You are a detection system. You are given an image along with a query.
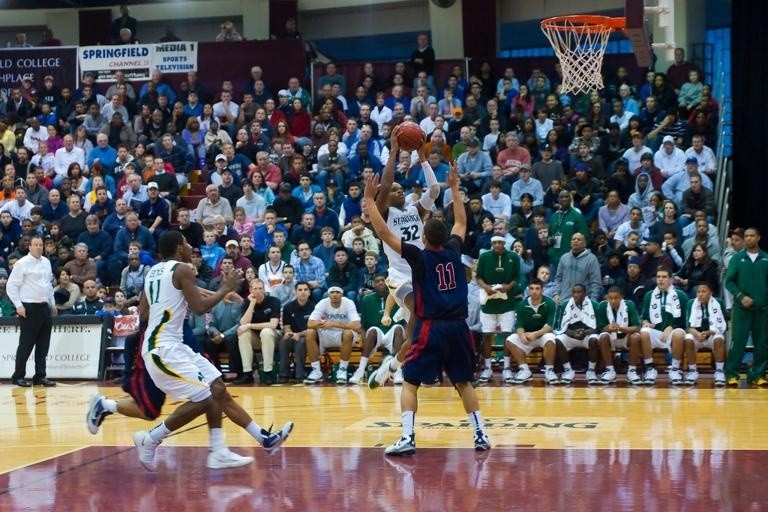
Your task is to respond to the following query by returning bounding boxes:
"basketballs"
[397,122,424,151]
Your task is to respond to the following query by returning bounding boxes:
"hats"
[729,228,745,239]
[643,235,662,244]
[686,156,697,164]
[148,182,158,189]
[412,179,422,186]
[520,164,531,172]
[226,240,239,248]
[663,135,674,145]
[465,140,479,146]
[215,154,226,162]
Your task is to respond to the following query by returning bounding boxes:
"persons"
[86,262,296,457]
[132,231,256,470]
[361,159,492,456]
[0,5,768,388]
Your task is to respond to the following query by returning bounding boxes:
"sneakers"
[233,375,254,385]
[475,431,490,451]
[261,422,295,457]
[383,433,415,455]
[368,354,404,389]
[133,430,157,472]
[86,393,112,434]
[752,378,768,385]
[350,371,366,382]
[263,370,347,384]
[479,366,739,386]
[206,449,254,469]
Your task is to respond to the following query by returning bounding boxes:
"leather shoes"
[33,378,55,386]
[12,375,30,387]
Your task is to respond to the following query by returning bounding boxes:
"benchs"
[85,342,728,385]
